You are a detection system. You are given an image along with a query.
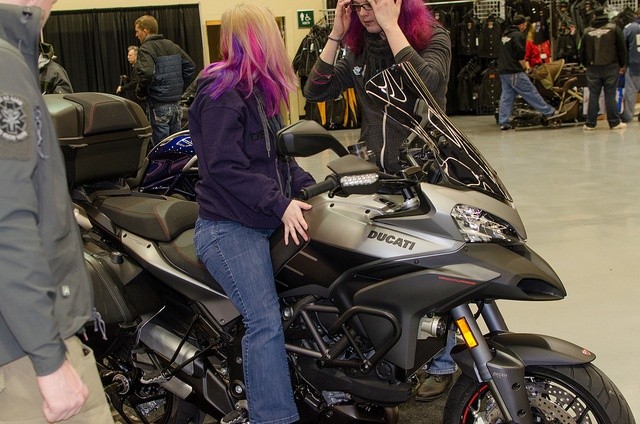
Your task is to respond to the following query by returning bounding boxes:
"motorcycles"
[36,88,639,421]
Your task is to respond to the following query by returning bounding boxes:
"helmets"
[138,135,195,193]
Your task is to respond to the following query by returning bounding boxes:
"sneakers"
[619,112,628,123]
[612,122,626,129]
[501,126,515,130]
[582,124,595,130]
[546,109,567,120]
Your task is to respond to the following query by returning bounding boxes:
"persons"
[133,14,196,144]
[302,0,452,176]
[495,15,567,130]
[621,8,640,123]
[579,6,628,129]
[525,16,551,72]
[116,45,147,114]
[186,3,312,424]
[1,0,117,423]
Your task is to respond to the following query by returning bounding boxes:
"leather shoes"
[415,374,453,402]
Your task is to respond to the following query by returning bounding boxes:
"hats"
[595,7,606,15]
[513,14,530,25]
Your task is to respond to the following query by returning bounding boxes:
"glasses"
[350,4,373,12]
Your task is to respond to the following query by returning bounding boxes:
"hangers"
[462,9,475,23]
[481,60,498,75]
[305,21,327,40]
[456,58,478,79]
